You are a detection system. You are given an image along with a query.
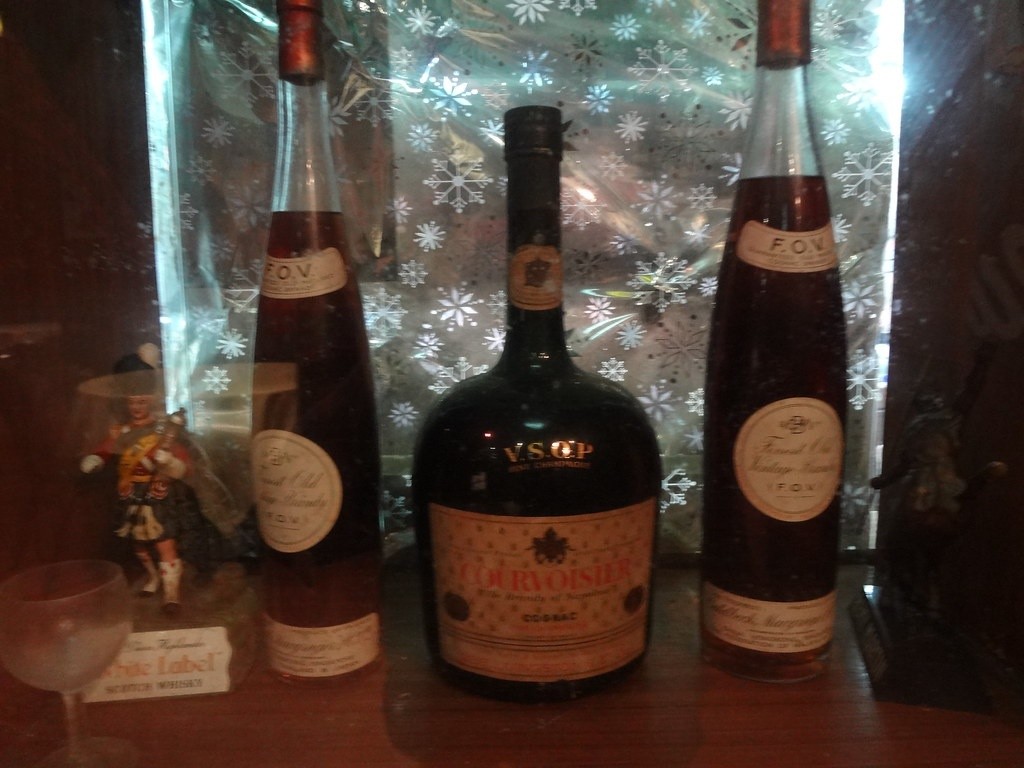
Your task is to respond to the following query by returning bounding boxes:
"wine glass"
[0,562,140,768]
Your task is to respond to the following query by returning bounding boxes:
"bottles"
[412,108,662,699]
[702,0,849,681]
[251,0,390,685]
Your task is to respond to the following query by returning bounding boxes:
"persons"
[79,351,191,614]
